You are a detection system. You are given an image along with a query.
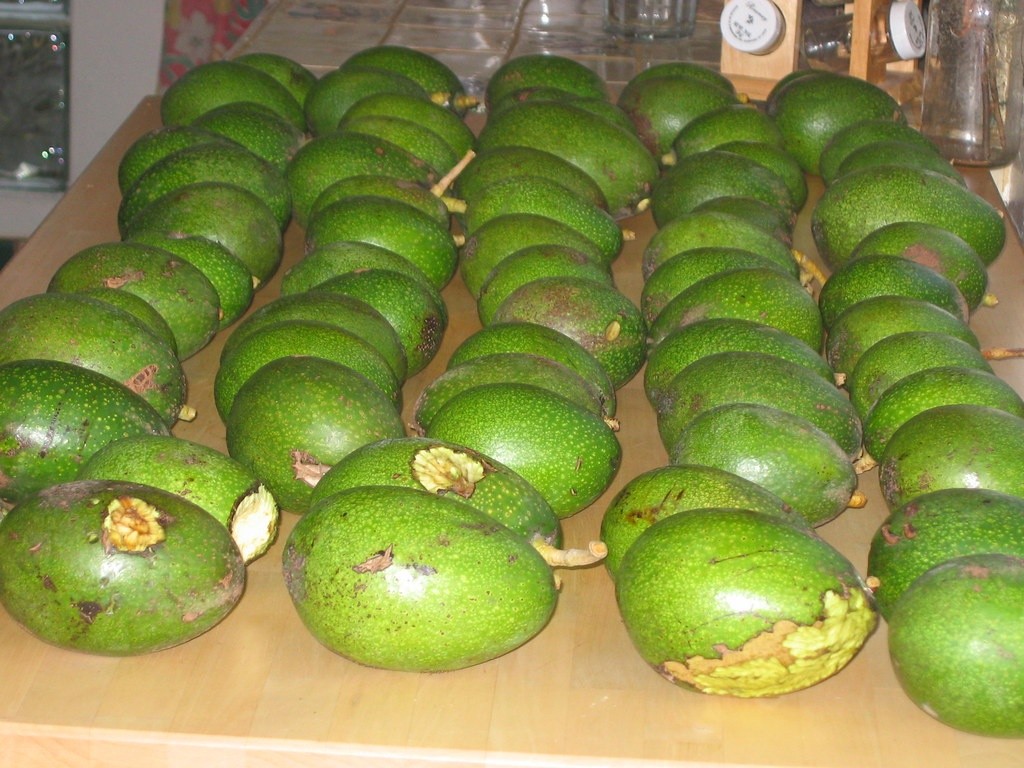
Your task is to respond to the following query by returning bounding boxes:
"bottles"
[795,0,925,75]
[919,0,1024,169]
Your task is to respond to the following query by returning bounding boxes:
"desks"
[0,86,1024,768]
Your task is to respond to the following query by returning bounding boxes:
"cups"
[608,0,698,43]
